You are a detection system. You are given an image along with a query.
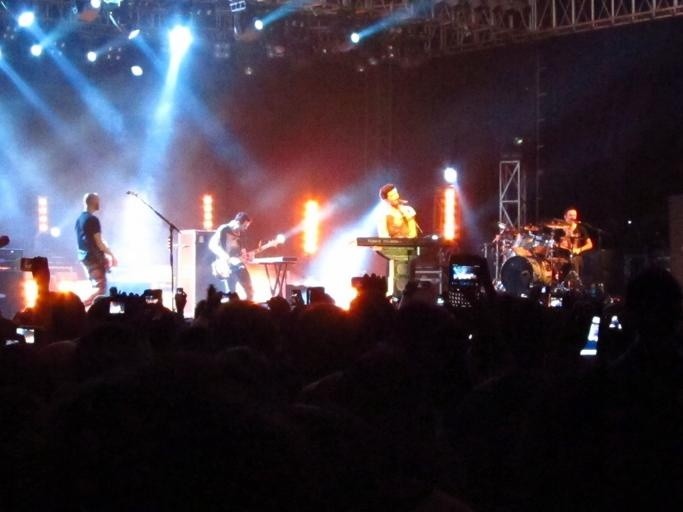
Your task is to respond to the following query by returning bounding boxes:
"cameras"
[218,294,232,304]
[392,296,401,303]
[435,296,446,304]
[550,295,564,308]
[144,294,160,305]
[606,313,624,331]
[14,326,36,346]
[107,298,127,316]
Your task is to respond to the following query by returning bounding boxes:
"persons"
[207,212,268,303]
[558,207,596,289]
[74,192,118,307]
[378,183,420,304]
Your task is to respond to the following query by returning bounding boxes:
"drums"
[511,234,541,257]
[529,235,555,256]
[500,256,559,297]
[546,246,571,264]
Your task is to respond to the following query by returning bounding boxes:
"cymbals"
[524,226,539,231]
[546,224,571,229]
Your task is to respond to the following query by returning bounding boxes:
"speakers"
[178,229,226,321]
[286,285,324,310]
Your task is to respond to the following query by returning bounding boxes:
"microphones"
[399,199,408,204]
[571,218,581,223]
[126,190,138,197]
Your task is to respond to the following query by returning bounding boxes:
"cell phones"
[578,314,603,358]
[352,277,371,287]
[21,258,34,271]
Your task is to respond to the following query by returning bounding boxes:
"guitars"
[211,234,286,280]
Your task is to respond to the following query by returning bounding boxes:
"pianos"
[356,236,460,247]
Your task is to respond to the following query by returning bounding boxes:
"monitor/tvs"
[449,260,485,287]
